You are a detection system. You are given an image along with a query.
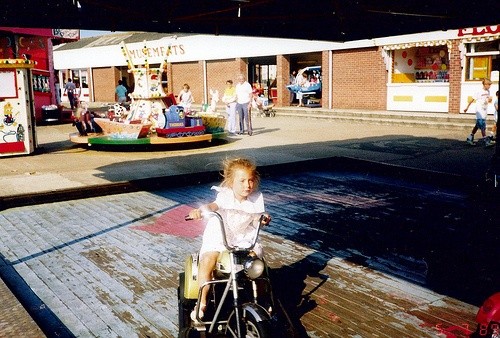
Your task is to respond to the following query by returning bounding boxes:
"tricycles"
[177,211,273,338]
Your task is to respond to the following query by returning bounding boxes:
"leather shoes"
[190,302,207,321]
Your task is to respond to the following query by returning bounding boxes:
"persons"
[222,79,238,135]
[177,83,195,111]
[463,77,496,147]
[115,80,128,103]
[64,78,78,109]
[233,73,253,133]
[491,89,500,140]
[188,156,273,323]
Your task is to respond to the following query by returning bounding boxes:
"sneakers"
[482,138,496,147]
[466,138,476,145]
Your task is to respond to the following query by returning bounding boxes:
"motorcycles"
[252,98,275,118]
